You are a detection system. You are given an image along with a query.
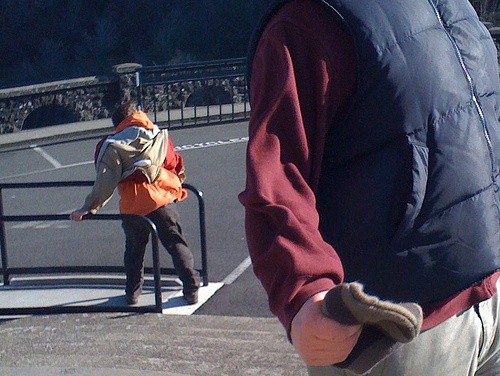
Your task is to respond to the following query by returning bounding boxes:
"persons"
[67,101,203,305]
[235,0,500,374]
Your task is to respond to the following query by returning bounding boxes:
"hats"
[323,281,425,345]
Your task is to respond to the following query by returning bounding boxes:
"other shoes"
[182,287,199,305]
[124,293,139,305]
